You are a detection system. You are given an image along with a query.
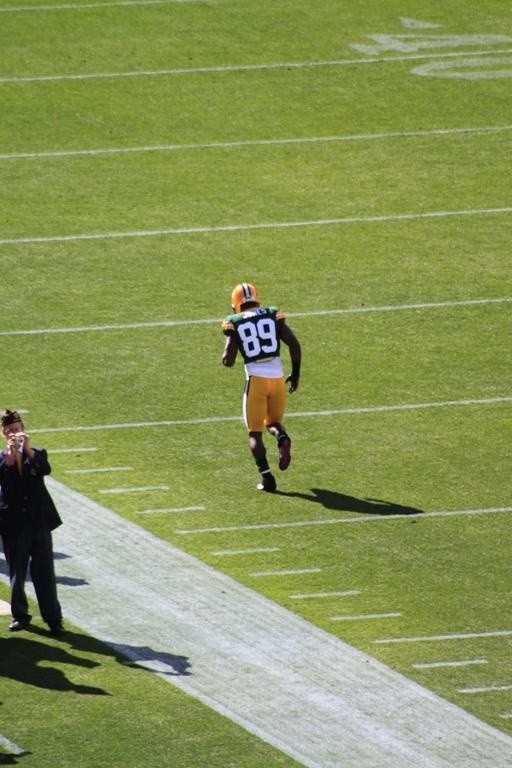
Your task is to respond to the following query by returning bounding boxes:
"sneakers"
[257,477,275,492]
[278,435,291,470]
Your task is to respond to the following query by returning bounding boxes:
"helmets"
[232,283,259,312]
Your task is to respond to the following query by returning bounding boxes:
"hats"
[1,410,20,426]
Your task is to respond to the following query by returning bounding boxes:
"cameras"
[14,432,25,446]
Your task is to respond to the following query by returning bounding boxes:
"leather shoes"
[8,615,32,630]
[48,618,63,635]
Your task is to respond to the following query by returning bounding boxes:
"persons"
[0,409,72,642]
[222,282,303,493]
